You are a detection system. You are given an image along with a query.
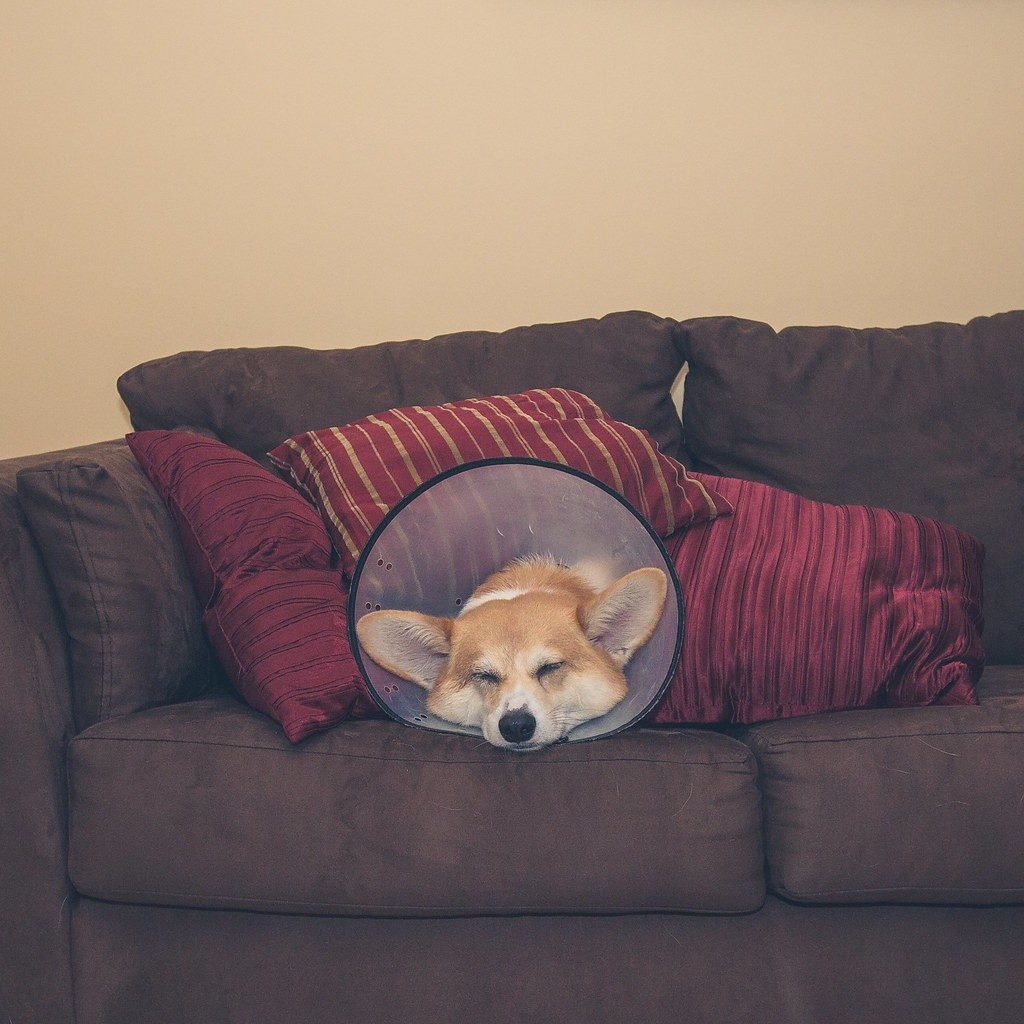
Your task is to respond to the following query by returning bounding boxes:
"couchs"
[0,301,1023,1024]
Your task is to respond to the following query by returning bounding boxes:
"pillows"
[262,385,738,602]
[630,465,981,727]
[123,426,387,746]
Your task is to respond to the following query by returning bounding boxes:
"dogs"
[354,551,668,752]
